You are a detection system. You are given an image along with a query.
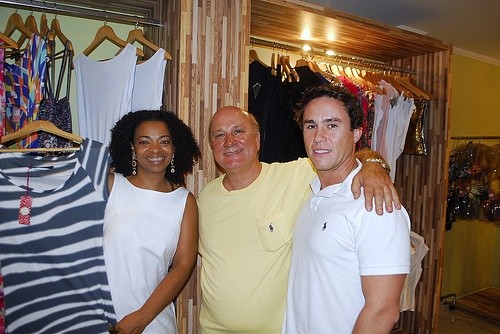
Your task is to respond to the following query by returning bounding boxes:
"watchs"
[363,157,391,173]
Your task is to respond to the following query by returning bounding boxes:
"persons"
[107,109,199,334]
[193,106,402,334]
[282,86,411,334]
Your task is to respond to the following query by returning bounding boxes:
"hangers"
[0,0,494,157]
